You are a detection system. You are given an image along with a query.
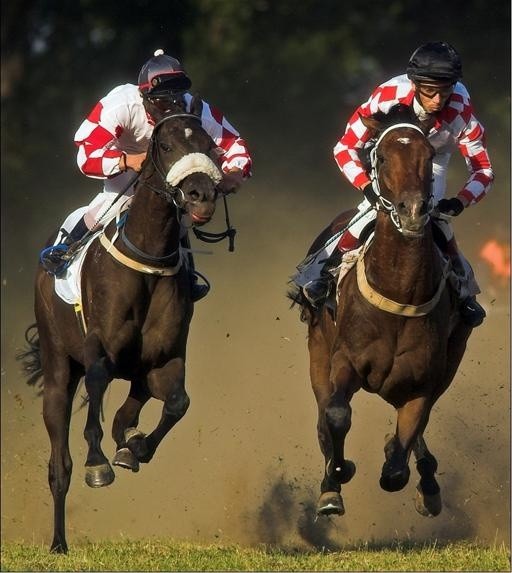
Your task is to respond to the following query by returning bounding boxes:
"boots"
[42,213,95,269]
[445,255,486,326]
[180,230,209,302]
[305,246,345,306]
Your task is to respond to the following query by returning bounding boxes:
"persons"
[300,42,499,330]
[38,49,252,302]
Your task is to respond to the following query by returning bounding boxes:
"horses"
[288,105,475,518]
[15,94,225,554]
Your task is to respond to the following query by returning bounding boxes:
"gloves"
[437,197,464,216]
[363,182,380,205]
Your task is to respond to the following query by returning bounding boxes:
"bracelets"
[123,153,129,172]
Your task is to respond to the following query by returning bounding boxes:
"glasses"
[414,81,457,98]
[147,97,185,110]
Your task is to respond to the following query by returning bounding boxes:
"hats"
[138,48,191,94]
[408,40,463,81]
